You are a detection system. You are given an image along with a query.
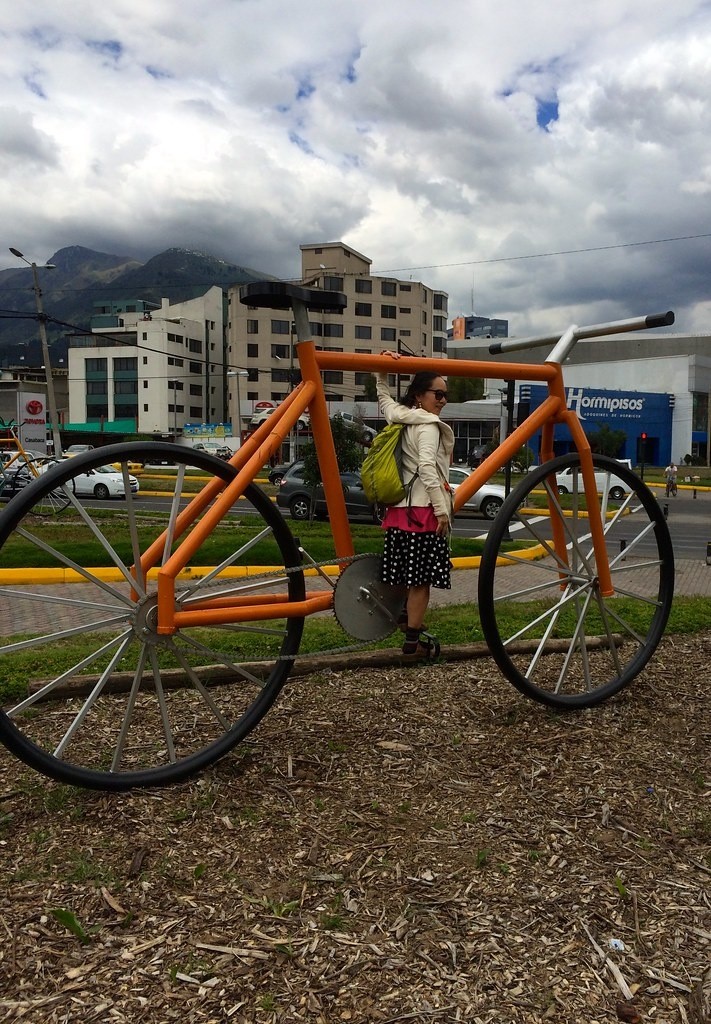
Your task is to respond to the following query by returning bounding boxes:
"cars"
[62,444,95,455]
[250,406,310,431]
[191,443,227,458]
[268,464,289,486]
[449,465,526,521]
[107,460,144,476]
[27,458,140,500]
[0,448,75,483]
[333,411,379,442]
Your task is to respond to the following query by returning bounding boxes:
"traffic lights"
[640,433,647,446]
[501,379,515,410]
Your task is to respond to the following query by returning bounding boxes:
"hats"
[669,462,674,466]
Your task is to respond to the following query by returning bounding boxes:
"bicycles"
[664,474,677,498]
[0,418,75,517]
[1,279,681,792]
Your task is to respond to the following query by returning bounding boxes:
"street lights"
[172,378,178,443]
[7,246,63,460]
[226,370,250,447]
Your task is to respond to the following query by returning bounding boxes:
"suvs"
[276,460,384,525]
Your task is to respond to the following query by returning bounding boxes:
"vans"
[556,458,633,500]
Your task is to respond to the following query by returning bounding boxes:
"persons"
[374,349,455,654]
[662,462,678,494]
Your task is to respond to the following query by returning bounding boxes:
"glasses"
[425,389,449,401]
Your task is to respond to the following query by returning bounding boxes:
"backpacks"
[359,423,423,529]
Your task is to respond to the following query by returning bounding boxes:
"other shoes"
[664,491,667,497]
[402,640,435,657]
